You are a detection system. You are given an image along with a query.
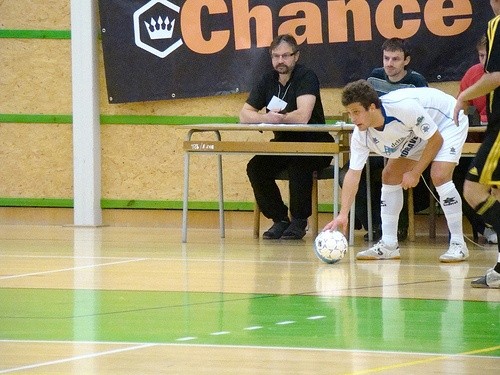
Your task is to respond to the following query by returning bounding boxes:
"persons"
[338,36,431,242]
[452,0,500,289]
[322,80,470,262]
[239,34,335,241]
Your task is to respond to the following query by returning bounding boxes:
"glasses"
[271,50,299,60]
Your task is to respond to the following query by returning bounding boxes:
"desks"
[175,124,342,244]
[342,123,488,246]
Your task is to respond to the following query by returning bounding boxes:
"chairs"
[253,113,479,241]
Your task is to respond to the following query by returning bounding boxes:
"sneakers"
[356,240,401,259]
[439,241,470,262]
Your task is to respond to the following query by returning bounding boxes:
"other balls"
[313,230,348,264]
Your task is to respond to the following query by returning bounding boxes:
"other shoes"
[364,225,408,243]
[484,227,498,244]
[263,218,290,239]
[281,222,308,239]
[471,265,500,289]
